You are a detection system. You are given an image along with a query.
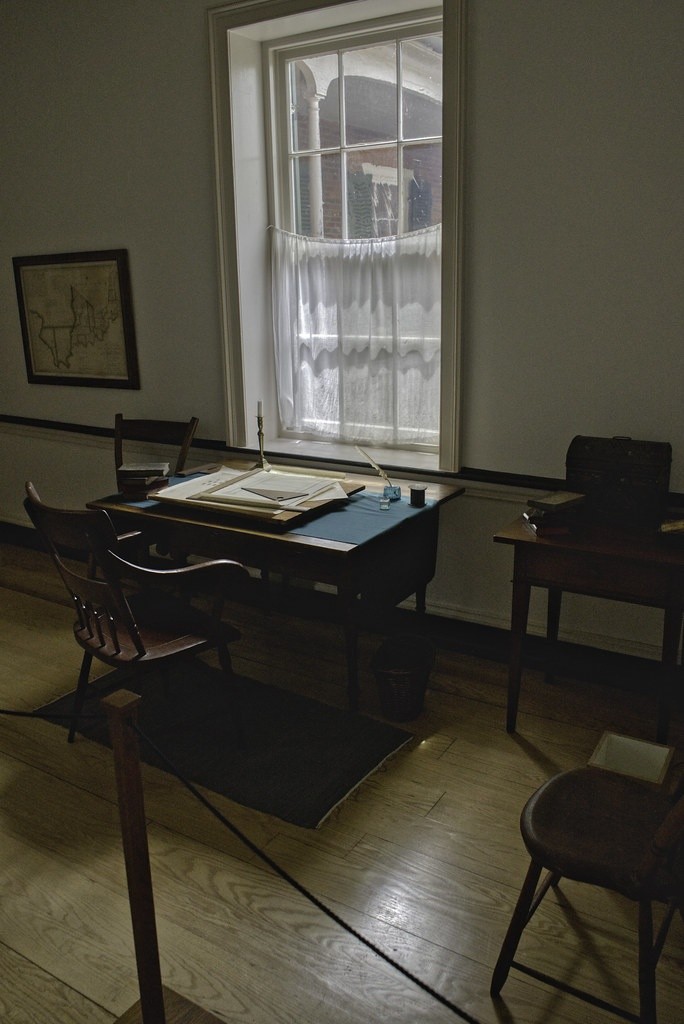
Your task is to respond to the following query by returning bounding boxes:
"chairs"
[115,413,200,486]
[487,768,684,1024]
[23,482,253,747]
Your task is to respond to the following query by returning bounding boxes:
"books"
[523,491,586,536]
[117,463,170,499]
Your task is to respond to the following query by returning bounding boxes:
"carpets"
[36,659,413,831]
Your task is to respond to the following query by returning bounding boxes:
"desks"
[88,457,466,746]
[492,505,684,734]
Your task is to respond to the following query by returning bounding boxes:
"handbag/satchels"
[564,435,673,507]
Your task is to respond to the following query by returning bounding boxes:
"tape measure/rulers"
[241,487,308,502]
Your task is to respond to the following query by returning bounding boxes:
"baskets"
[368,634,436,722]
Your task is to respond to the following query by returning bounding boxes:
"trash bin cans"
[370,633,437,721]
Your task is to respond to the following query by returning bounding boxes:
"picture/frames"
[11,249,141,391]
